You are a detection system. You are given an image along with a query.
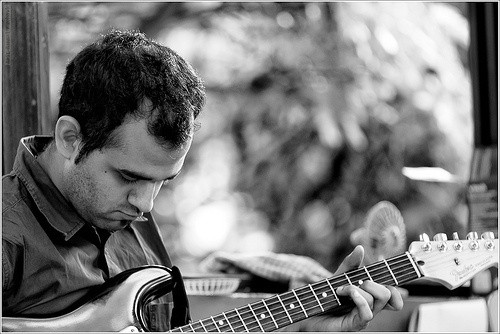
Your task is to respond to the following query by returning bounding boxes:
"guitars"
[19,232,499,333]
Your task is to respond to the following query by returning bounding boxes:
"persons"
[2,28,408,332]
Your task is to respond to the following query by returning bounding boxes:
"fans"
[348,199,410,266]
